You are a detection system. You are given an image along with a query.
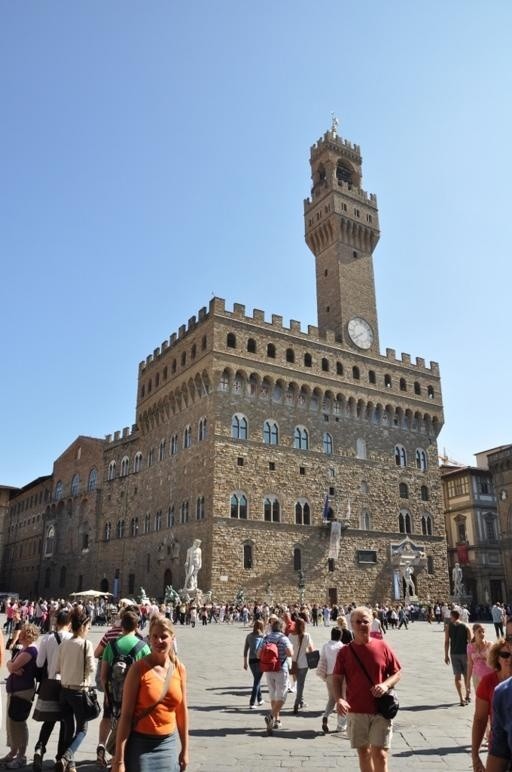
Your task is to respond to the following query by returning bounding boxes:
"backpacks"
[258,642,281,673]
[107,638,147,706]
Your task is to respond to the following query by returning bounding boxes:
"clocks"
[348,315,375,351]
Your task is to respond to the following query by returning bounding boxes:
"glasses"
[497,650,512,659]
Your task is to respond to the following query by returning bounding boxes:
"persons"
[402,561,415,596]
[453,562,462,595]
[184,539,202,588]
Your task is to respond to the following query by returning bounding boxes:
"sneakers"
[264,714,284,735]
[321,716,331,735]
[258,699,265,705]
[54,757,77,772]
[459,697,471,707]
[248,704,258,709]
[0,755,27,769]
[292,702,309,714]
[33,748,42,772]
[94,743,108,771]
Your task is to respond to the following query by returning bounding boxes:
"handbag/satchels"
[8,695,32,722]
[305,650,320,670]
[374,688,400,720]
[317,656,329,681]
[32,677,63,723]
[289,660,299,675]
[79,688,101,722]
